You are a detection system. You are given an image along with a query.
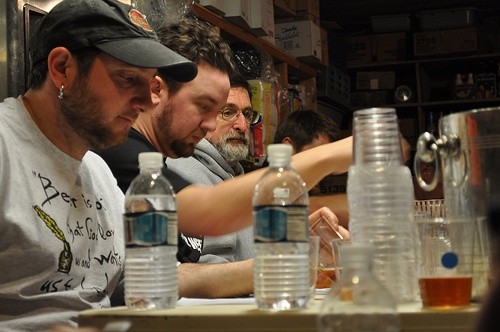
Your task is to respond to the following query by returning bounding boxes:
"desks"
[76,300,484,332]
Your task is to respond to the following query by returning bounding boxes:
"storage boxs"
[195,0,499,168]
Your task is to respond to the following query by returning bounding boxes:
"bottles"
[251,143,312,312]
[123,151,178,311]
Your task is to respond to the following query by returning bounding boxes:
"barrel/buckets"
[415,107,500,301]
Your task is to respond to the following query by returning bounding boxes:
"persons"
[93,18,411,261]
[0,0,312,332]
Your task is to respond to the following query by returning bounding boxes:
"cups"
[308,236,319,298]
[414,211,476,311]
[330,238,372,302]
[345,108,416,305]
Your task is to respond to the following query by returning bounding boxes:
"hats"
[28,0,197,83]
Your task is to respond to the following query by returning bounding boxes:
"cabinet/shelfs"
[344,6,500,151]
[191,4,320,174]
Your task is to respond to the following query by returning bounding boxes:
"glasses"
[219,108,262,125]
